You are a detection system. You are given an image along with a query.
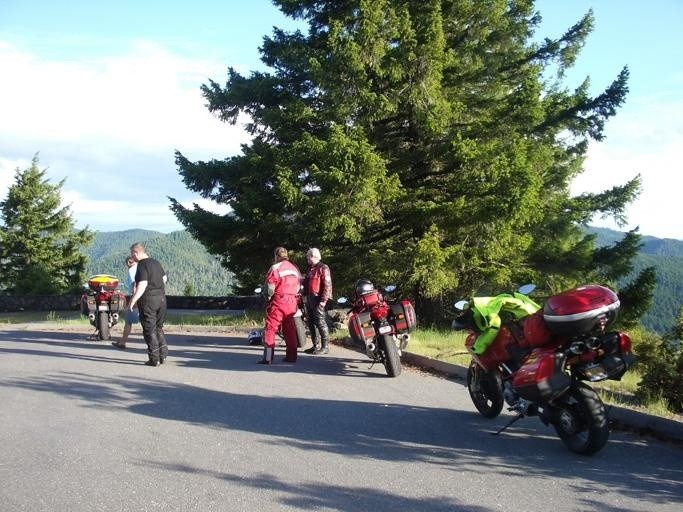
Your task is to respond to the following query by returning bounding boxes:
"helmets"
[248,330,262,345]
[356,279,374,297]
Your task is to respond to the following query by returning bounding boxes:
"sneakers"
[313,346,329,354]
[305,347,319,353]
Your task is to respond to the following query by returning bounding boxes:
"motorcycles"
[454,283,637,456]
[255,285,306,347]
[337,279,416,376]
[81,274,126,341]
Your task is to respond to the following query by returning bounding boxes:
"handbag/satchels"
[524,308,554,347]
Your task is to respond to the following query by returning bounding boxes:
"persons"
[302,248,333,354]
[112,256,138,349]
[129,243,168,367]
[257,247,302,364]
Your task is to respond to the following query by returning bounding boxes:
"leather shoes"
[282,357,296,363]
[111,341,125,349]
[145,358,166,366]
[256,359,271,364]
